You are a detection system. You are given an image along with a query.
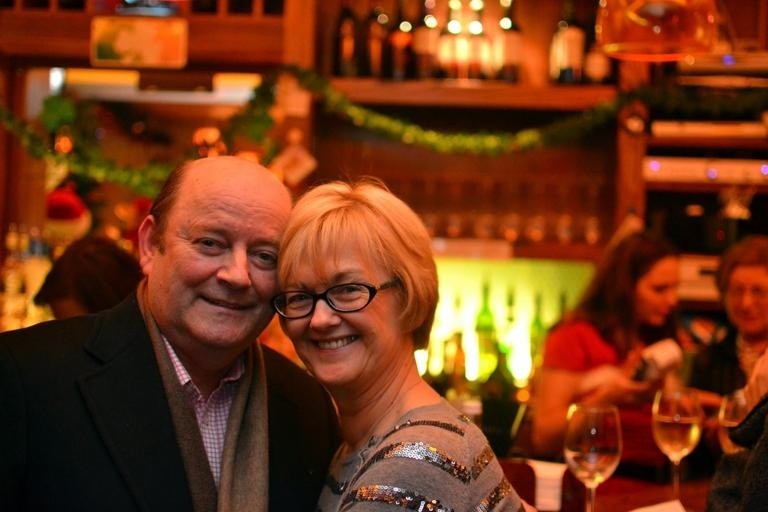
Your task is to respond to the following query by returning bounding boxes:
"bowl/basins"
[674,76,764,120]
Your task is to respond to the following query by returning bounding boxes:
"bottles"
[439,285,570,382]
[333,0,611,84]
[0,226,73,325]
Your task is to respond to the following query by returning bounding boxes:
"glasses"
[272,278,401,318]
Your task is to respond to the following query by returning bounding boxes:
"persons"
[680,236,768,512]
[0,156,342,512]
[530,234,732,456]
[277,180,525,512]
[34,235,140,320]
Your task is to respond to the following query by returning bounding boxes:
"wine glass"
[562,387,705,512]
